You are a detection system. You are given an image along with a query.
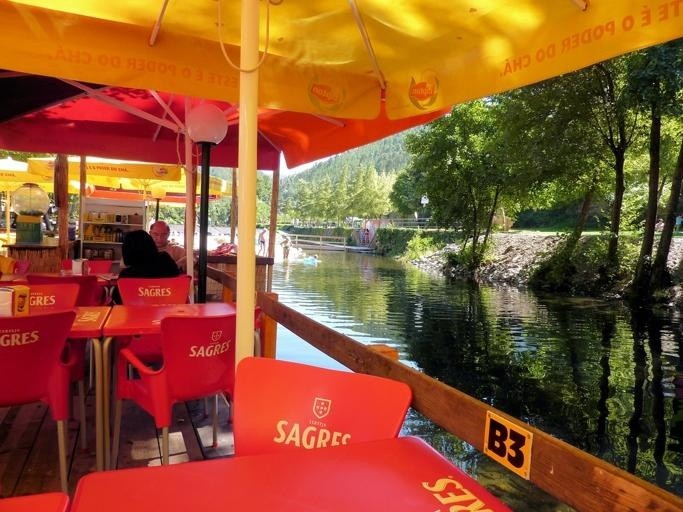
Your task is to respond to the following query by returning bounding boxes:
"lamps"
[185,103,228,304]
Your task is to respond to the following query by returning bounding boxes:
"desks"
[31,305,112,474]
[102,303,237,470]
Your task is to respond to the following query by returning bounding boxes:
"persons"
[279,235,292,260]
[358,227,364,245]
[148,221,197,272]
[303,254,318,261]
[256,228,267,257]
[363,228,370,245]
[110,230,181,304]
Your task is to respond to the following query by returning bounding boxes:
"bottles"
[115,210,121,224]
[68,216,76,240]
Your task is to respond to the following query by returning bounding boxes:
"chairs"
[231,355,414,455]
[110,305,263,470]
[1,260,193,308]
[2,310,86,494]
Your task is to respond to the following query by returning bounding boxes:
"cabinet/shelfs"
[80,196,146,264]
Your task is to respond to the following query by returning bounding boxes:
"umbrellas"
[0,0,683,369]
[28,156,232,199]
[1,70,453,305]
[0,156,94,243]
[86,185,222,224]
